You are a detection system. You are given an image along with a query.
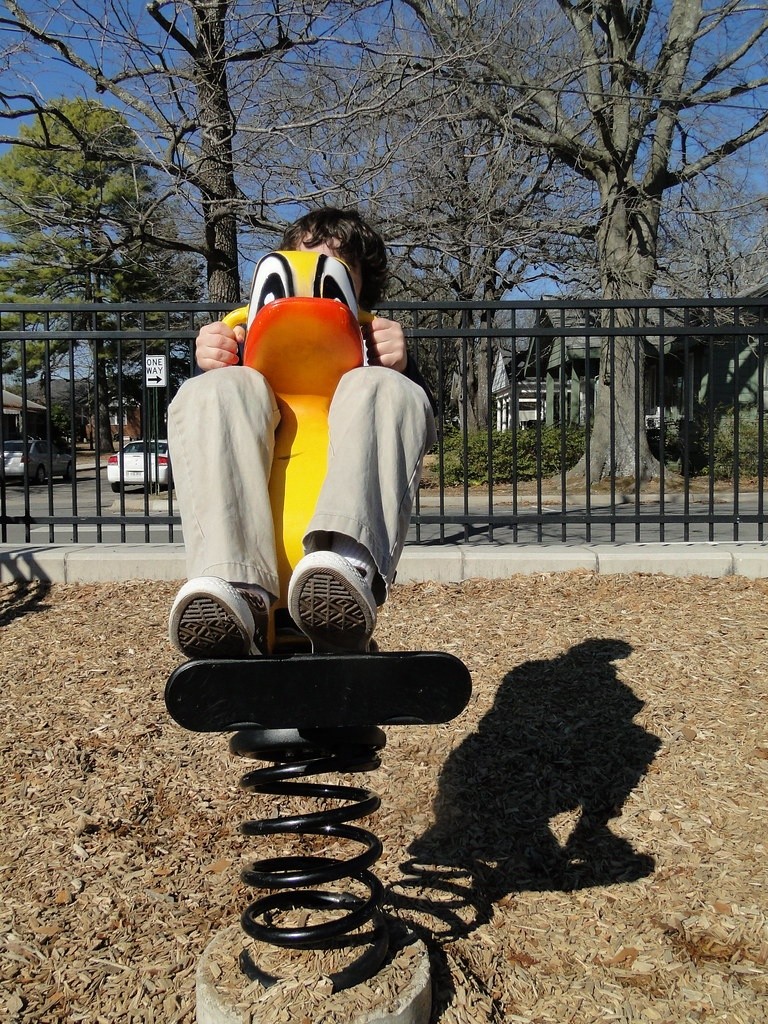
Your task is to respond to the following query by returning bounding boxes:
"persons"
[165,205,439,661]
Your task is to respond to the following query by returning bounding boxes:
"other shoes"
[168,577,268,657]
[287,551,377,656]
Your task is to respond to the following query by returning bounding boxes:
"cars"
[107,439,174,492]
[4,440,73,484]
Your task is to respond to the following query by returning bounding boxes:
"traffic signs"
[146,354,166,387]
